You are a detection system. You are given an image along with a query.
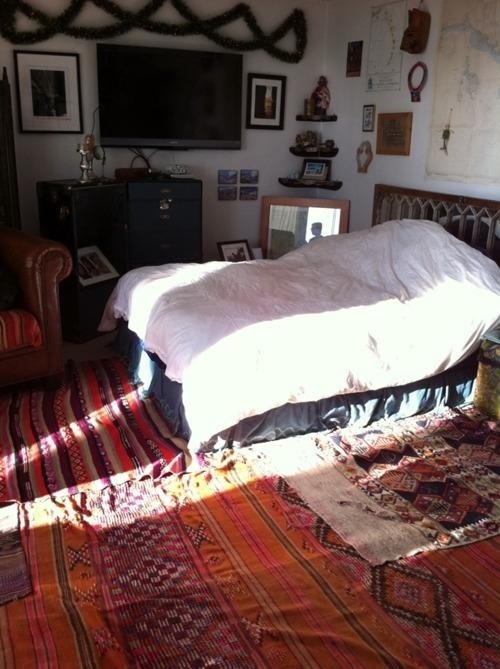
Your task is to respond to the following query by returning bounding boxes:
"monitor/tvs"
[96,42,242,149]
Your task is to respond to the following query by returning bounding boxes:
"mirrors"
[259,194,352,258]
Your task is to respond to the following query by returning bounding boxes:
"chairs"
[1,225,74,393]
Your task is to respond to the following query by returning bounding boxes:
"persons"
[307,221,325,243]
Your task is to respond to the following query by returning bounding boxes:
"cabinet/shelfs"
[276,110,344,192]
[33,177,130,344]
[123,174,204,269]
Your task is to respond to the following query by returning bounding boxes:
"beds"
[97,183,500,463]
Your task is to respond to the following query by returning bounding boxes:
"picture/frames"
[12,48,84,134]
[374,109,413,158]
[362,102,375,133]
[300,156,331,182]
[245,72,287,130]
[216,239,255,265]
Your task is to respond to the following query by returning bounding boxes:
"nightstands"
[471,337,500,423]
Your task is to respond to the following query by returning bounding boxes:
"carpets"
[239,402,499,568]
